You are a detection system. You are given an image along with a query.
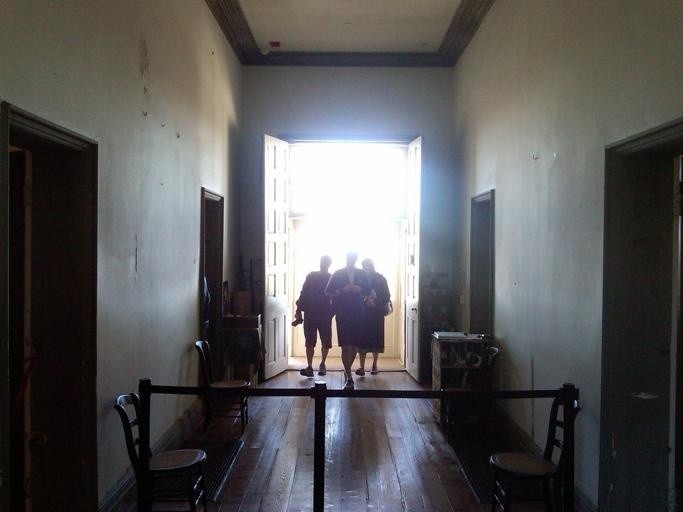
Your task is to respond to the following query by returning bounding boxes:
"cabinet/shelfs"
[431,335,497,430]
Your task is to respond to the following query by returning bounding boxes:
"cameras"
[291,319,303,327]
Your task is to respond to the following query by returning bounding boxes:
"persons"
[295,252,390,390]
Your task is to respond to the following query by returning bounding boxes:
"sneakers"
[371,367,378,374]
[343,370,354,390]
[355,367,366,377]
[299,366,314,378]
[317,364,326,375]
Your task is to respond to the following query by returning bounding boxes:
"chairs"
[441,344,500,445]
[194,338,251,435]
[488,382,582,512]
[113,392,207,512]
